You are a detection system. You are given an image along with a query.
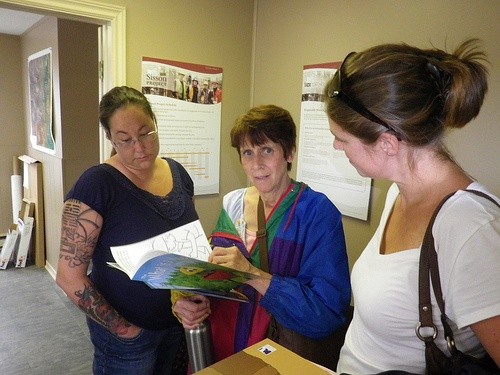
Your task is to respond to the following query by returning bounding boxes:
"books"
[104,218,273,304]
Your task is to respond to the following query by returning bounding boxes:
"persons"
[172,73,223,105]
[319,42,500,375]
[175,105,351,366]
[55,86,201,375]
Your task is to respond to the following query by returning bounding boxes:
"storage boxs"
[18,155,44,268]
[0,223,20,270]
[14,198,34,268]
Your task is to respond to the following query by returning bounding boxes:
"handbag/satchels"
[414,188,500,374]
[269,305,354,372]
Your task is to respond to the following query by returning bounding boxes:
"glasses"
[106,116,159,150]
[327,51,404,143]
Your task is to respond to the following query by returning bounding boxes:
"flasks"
[187,300,215,374]
